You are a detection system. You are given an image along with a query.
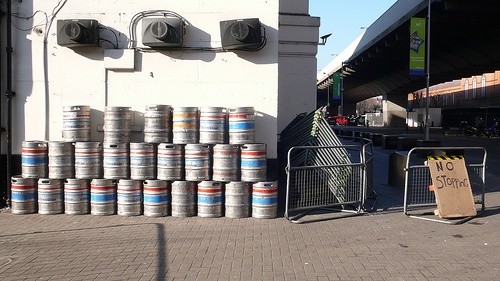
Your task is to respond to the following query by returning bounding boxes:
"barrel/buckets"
[241,142,267,182]
[117,179,142,217]
[89,179,117,216]
[143,180,169,216]
[74,141,102,179]
[172,106,199,144]
[64,179,90,215]
[144,104,174,144]
[170,181,198,217]
[37,179,63,215]
[11,175,37,214]
[103,106,133,143]
[21,140,48,178]
[129,142,156,180]
[211,142,240,183]
[199,107,227,144]
[156,142,183,181]
[226,106,255,145]
[48,141,75,179]
[102,142,129,179]
[224,181,251,219]
[251,181,278,219]
[198,181,224,218]
[185,144,210,181]
[61,105,91,142]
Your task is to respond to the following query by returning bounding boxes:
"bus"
[327,116,349,126]
